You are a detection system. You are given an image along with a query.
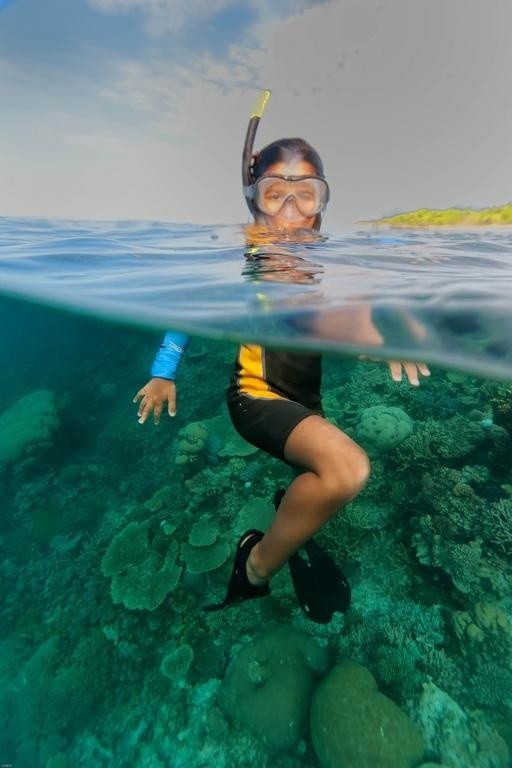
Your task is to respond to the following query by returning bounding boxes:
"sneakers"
[232,530,269,599]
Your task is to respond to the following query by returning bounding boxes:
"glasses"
[253,176,329,218]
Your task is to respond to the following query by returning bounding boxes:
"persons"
[129,135,431,623]
